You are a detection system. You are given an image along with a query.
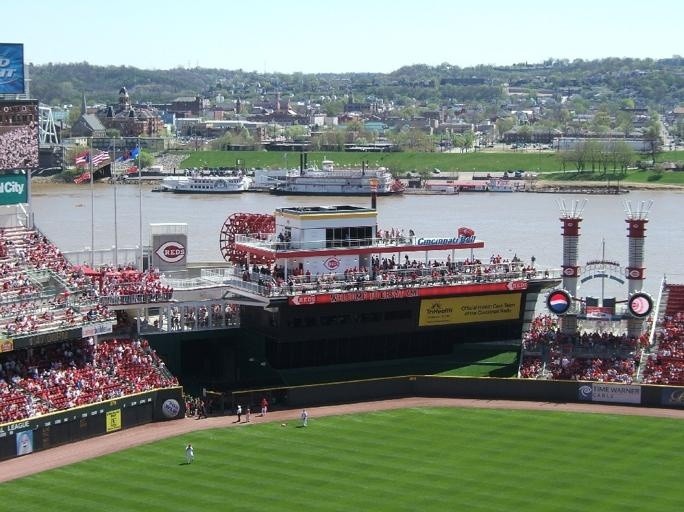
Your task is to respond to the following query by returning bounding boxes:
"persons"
[176,376,208,420]
[184,443,194,464]
[301,409,307,426]
[115,304,241,333]
[239,229,535,297]
[259,396,268,416]
[244,404,252,423]
[0,229,115,339]
[81,261,174,304]
[0,337,179,426]
[520,309,683,388]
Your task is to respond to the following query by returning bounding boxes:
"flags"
[72,146,138,184]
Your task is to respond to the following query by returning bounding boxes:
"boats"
[163,153,392,195]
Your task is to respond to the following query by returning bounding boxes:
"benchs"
[0,226,180,425]
[287,259,533,296]
[519,281,684,387]
